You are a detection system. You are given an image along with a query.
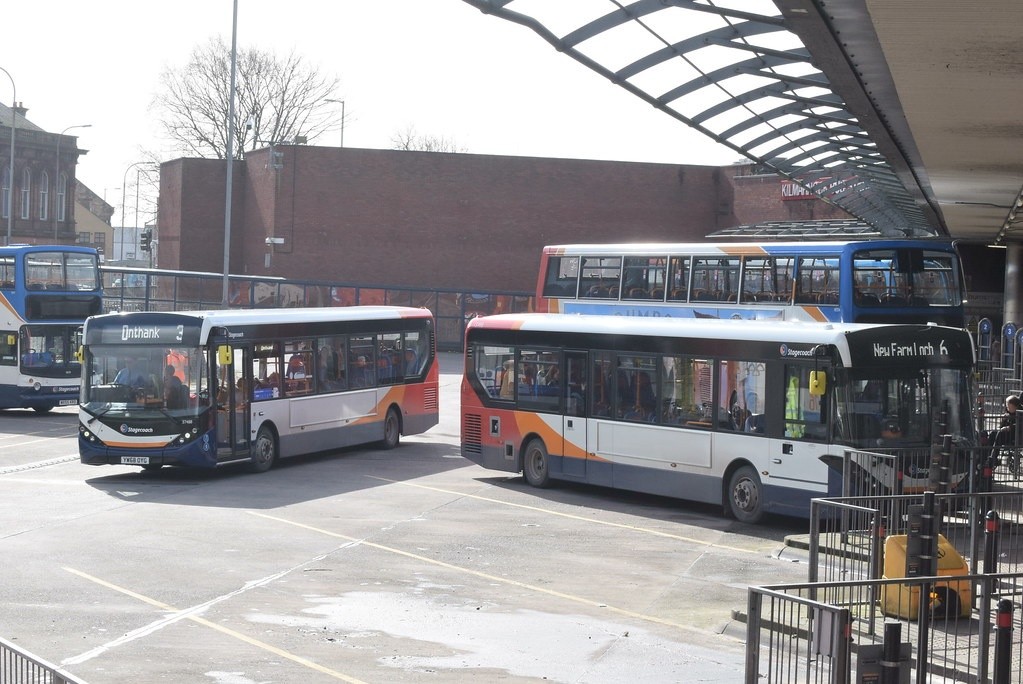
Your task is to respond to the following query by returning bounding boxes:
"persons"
[164,365,183,410]
[113,356,144,387]
[980,389,1023,460]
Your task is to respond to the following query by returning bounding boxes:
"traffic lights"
[141,230,152,251]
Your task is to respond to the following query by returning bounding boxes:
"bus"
[0,243,105,412]
[461,312,979,524]
[73,305,439,473]
[535,240,967,424]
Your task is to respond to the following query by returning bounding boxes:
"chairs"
[494,360,765,432]
[237,348,416,391]
[0,281,78,290]
[543,283,932,305]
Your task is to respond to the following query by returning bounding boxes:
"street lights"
[324,99,344,147]
[121,162,155,261]
[54,125,92,245]
